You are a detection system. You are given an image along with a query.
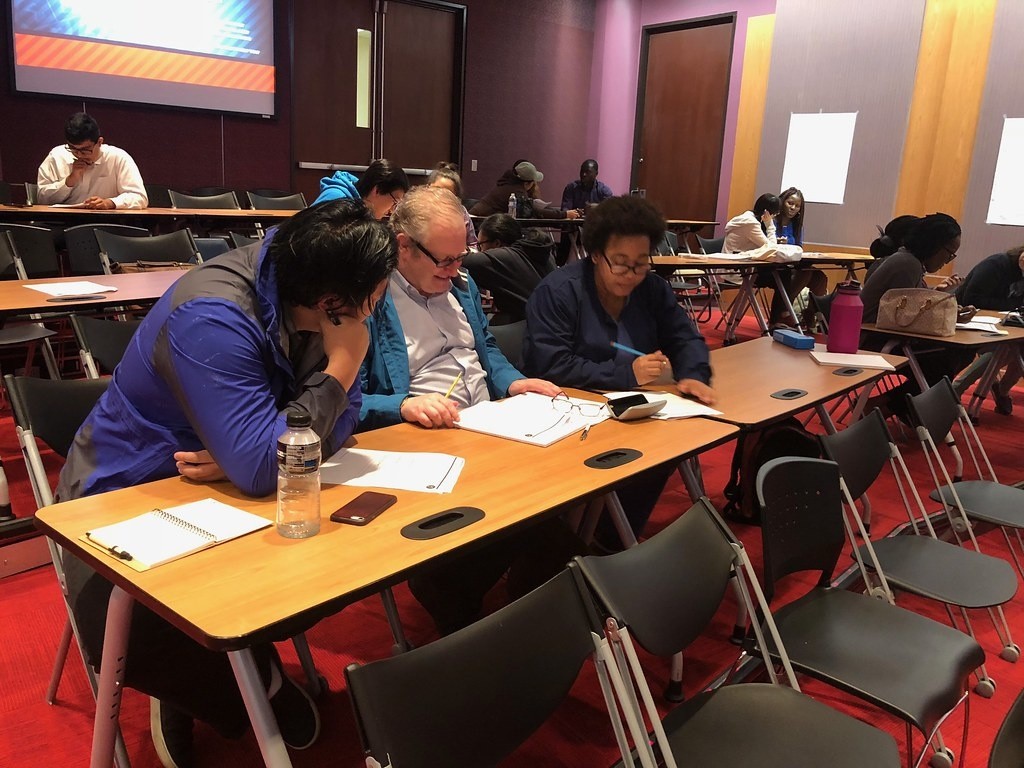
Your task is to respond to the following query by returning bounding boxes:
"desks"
[468,215,588,261]
[0,204,302,223]
[32,375,743,768]
[664,220,721,294]
[0,269,192,316]
[647,256,775,341]
[860,307,1024,425]
[594,334,911,645]
[800,252,873,328]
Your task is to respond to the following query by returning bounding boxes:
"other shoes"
[990,381,1013,416]
[853,397,872,421]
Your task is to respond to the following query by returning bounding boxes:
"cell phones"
[330,492,397,526]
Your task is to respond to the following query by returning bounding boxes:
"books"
[85,496,274,574]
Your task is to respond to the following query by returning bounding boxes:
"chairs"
[0,178,1024,768]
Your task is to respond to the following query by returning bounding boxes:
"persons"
[462,214,553,326]
[557,160,613,266]
[308,159,407,221]
[514,195,715,558]
[54,196,398,768]
[353,186,564,640]
[720,188,828,332]
[468,159,579,231]
[36,110,150,210]
[860,213,1024,427]
[427,160,477,253]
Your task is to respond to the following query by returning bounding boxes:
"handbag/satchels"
[876,288,958,337]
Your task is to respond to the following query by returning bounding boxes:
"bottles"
[277,411,321,539]
[827,280,864,353]
[509,193,516,220]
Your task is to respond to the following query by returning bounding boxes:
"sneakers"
[267,642,321,749]
[149,696,194,768]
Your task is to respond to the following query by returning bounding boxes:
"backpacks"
[722,416,822,525]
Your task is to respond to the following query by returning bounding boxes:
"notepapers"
[997,330,1009,335]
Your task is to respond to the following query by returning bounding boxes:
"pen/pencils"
[760,209,767,222]
[580,423,591,441]
[325,309,341,326]
[73,158,91,165]
[686,261,708,263]
[444,371,464,398]
[85,532,133,562]
[610,341,667,364]
[959,309,981,316]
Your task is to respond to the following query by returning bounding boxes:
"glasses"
[552,391,605,417]
[476,240,488,252]
[65,141,96,156]
[408,236,470,269]
[388,192,400,207]
[601,251,655,276]
[943,246,957,264]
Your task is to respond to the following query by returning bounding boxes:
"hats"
[515,162,544,182]
